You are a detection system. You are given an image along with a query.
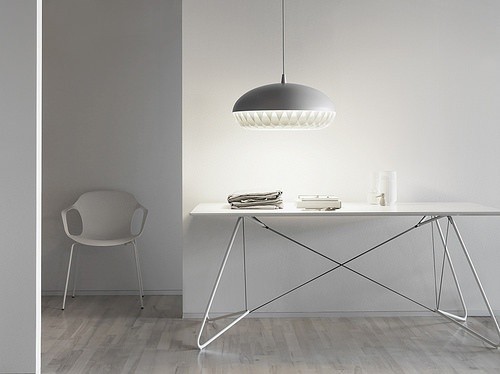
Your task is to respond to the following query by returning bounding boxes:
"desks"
[191,201,500,349]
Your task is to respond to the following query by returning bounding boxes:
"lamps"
[230,2,338,130]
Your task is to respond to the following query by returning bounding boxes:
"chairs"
[61,189,150,310]
